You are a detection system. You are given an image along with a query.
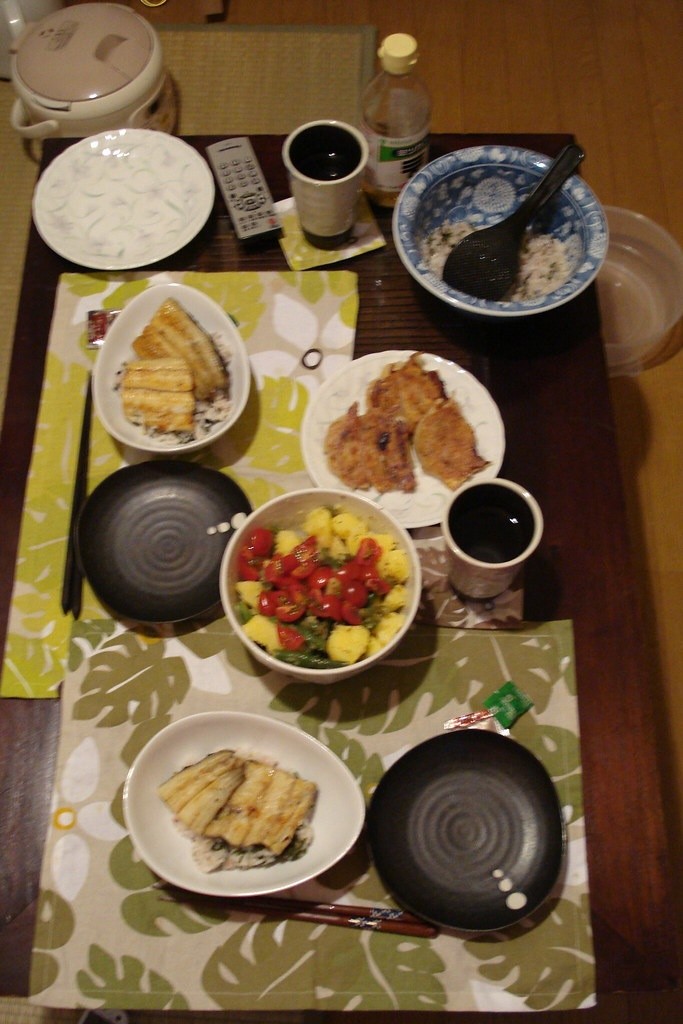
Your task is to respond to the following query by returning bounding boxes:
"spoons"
[442,144,586,302]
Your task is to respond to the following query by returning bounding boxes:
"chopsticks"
[62,370,93,621]
[153,883,440,938]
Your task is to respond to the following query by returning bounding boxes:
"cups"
[0,0,60,79]
[440,478,543,599]
[283,119,370,236]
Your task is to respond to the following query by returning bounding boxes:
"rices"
[420,219,573,300]
[112,328,233,447]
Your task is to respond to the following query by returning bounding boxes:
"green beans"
[238,606,350,668]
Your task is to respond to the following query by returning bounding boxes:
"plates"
[300,351,506,527]
[31,128,215,270]
[369,729,563,931]
[78,461,252,622]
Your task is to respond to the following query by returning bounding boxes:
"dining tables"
[0,128,682,1024]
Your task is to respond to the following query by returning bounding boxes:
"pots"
[8,3,176,139]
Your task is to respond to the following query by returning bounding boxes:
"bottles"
[358,34,430,206]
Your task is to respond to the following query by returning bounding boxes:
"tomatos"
[238,528,391,649]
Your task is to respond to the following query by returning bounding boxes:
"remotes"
[206,135,283,243]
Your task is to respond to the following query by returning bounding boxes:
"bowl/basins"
[122,710,366,896]
[92,284,251,452]
[218,488,420,684]
[596,205,682,378]
[392,145,608,316]
